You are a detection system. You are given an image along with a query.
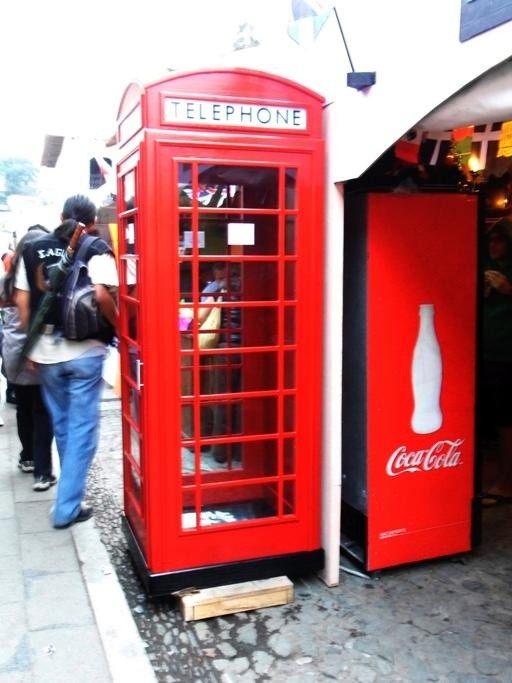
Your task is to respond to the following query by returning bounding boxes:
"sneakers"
[21,459,57,491]
[207,459,240,471]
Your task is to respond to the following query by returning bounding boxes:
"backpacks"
[62,235,111,340]
[180,296,222,349]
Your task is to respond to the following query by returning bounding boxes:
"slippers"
[481,492,512,508]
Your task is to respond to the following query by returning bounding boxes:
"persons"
[200,259,226,302]
[199,261,242,463]
[477,216,510,507]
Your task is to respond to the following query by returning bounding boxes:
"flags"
[286,0,336,45]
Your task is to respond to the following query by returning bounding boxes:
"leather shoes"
[54,506,93,529]
